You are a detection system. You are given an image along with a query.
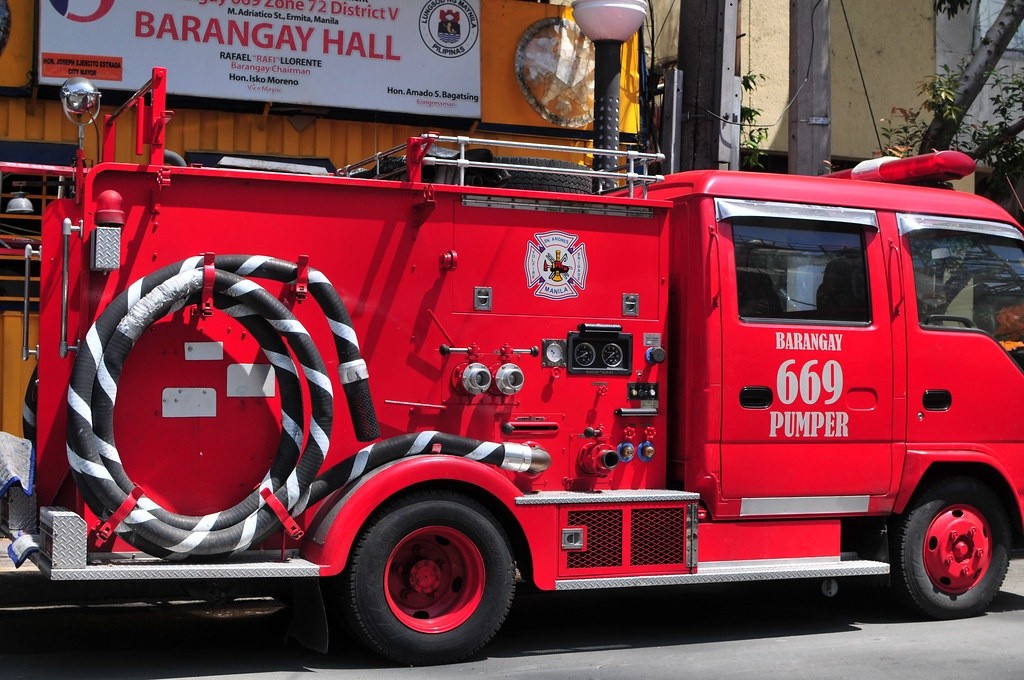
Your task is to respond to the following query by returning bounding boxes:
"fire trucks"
[1,66,1023,670]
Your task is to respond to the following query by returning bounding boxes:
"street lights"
[571,0,650,192]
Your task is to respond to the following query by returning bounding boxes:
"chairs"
[739,271,788,318]
[815,256,868,322]
